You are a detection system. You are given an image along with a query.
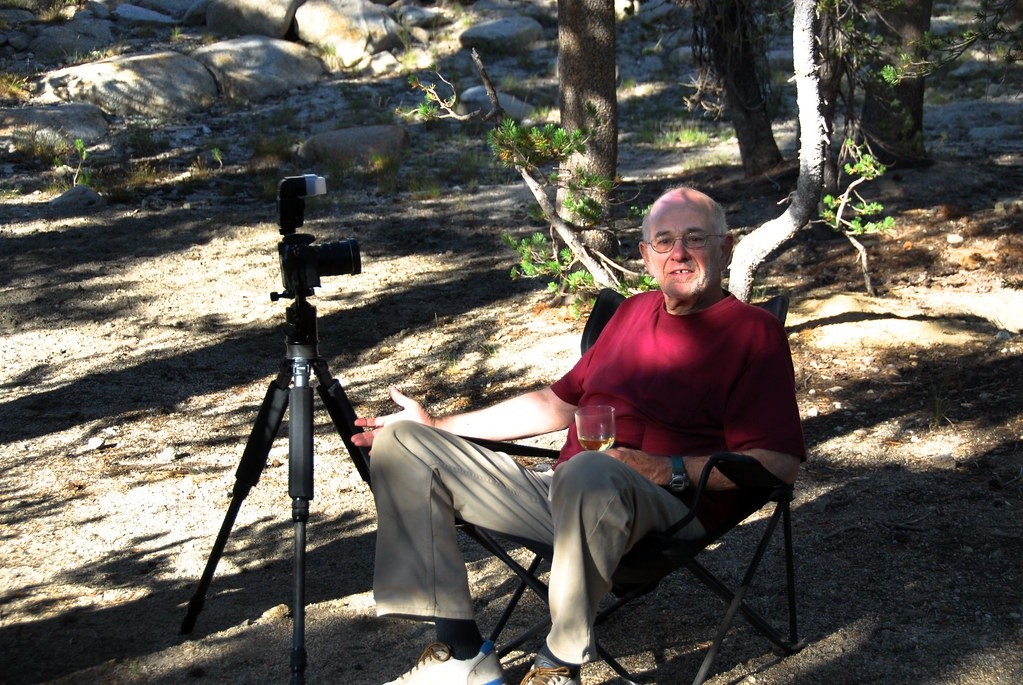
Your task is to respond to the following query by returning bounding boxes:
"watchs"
[667,453,691,493]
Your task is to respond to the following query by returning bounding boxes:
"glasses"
[645,231,725,254]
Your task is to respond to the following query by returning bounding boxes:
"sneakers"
[382,640,506,685]
[519,664,582,685]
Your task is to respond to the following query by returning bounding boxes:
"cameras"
[277,174,362,291]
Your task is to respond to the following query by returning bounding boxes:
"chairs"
[454,287,806,685]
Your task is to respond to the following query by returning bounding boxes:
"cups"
[574,405,616,452]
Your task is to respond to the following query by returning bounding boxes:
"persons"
[348,186,808,685]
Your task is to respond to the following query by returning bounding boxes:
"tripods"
[181,290,373,685]
